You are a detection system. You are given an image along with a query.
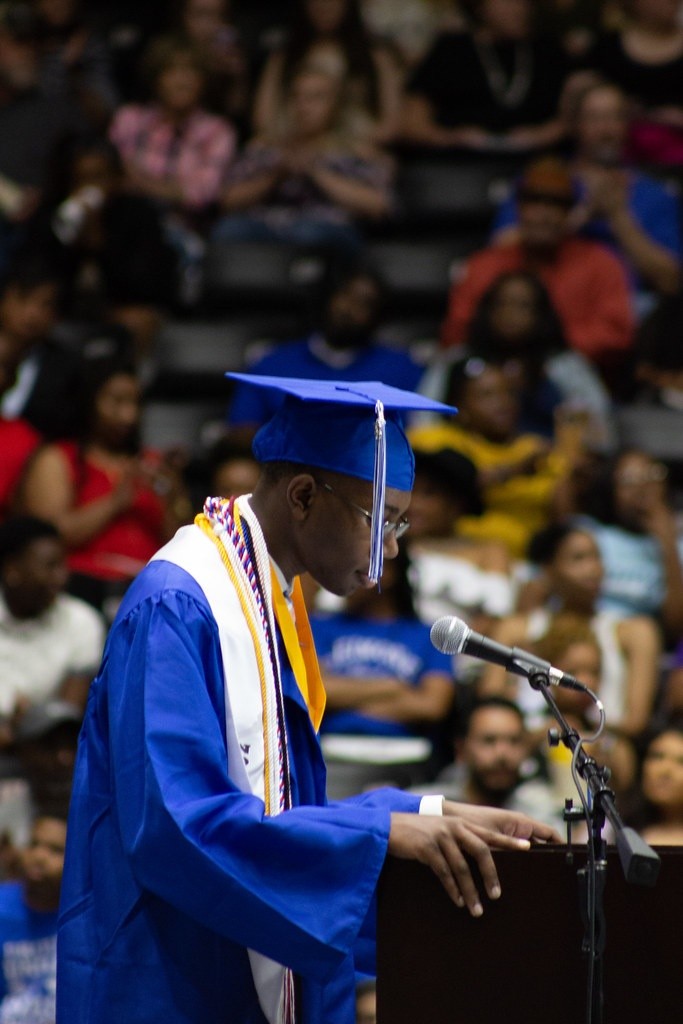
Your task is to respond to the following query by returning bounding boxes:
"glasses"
[317,478,411,540]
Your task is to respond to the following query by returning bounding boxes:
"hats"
[224,371,458,578]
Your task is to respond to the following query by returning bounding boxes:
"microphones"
[431,615,589,692]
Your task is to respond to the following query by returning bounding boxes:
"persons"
[56,373,566,1024]
[0,0,683,1024]
